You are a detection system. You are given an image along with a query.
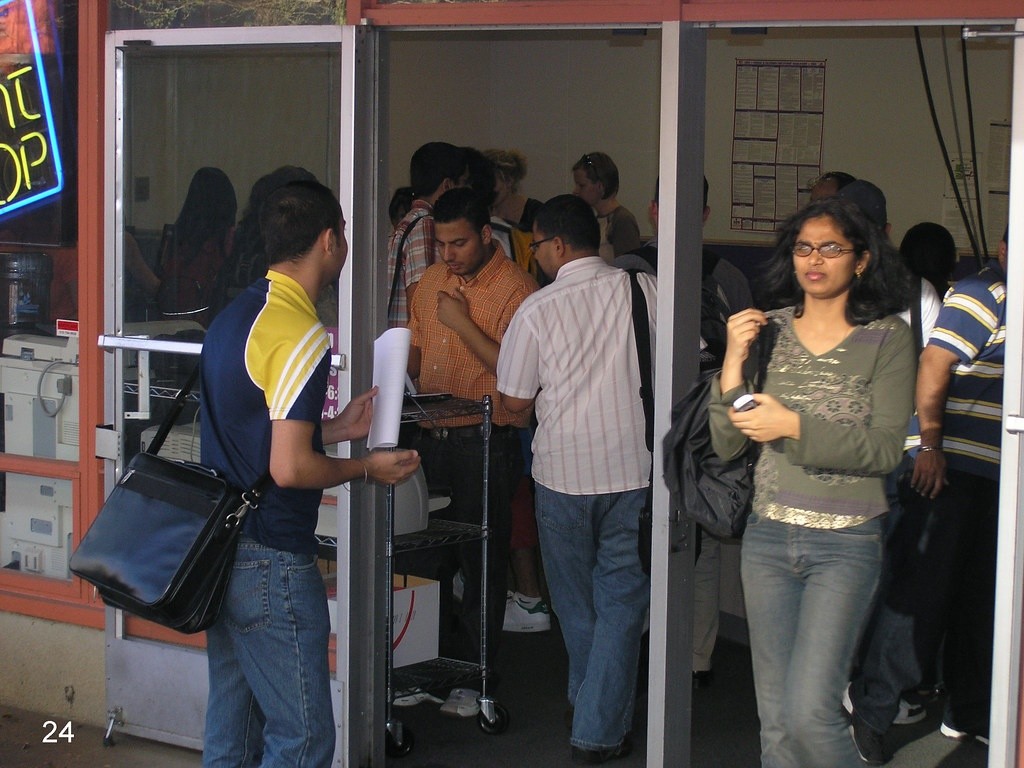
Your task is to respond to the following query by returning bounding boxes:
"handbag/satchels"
[69,453,244,635]
[662,320,769,538]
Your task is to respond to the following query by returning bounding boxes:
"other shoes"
[571,736,632,764]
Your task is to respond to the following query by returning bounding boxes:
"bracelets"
[916,445,943,452]
[343,459,367,492]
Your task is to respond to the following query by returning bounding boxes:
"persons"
[496,197,709,767]
[199,182,421,768]
[641,171,721,690]
[572,152,641,262]
[807,172,959,765]
[709,199,917,768]
[384,139,551,632]
[45,223,154,327]
[390,191,538,715]
[151,165,336,328]
[911,225,1006,745]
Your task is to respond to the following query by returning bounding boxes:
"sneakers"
[500,591,550,631]
[847,710,886,765]
[392,687,443,706]
[842,681,927,723]
[439,688,481,718]
[940,722,989,745]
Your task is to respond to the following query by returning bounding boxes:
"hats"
[839,180,887,226]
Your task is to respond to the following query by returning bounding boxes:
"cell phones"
[732,394,758,413]
[405,394,453,404]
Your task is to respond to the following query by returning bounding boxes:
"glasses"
[529,237,554,252]
[789,242,855,259]
[581,155,597,172]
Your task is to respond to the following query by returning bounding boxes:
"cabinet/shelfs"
[386,391,495,755]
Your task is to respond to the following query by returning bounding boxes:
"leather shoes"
[692,671,710,688]
